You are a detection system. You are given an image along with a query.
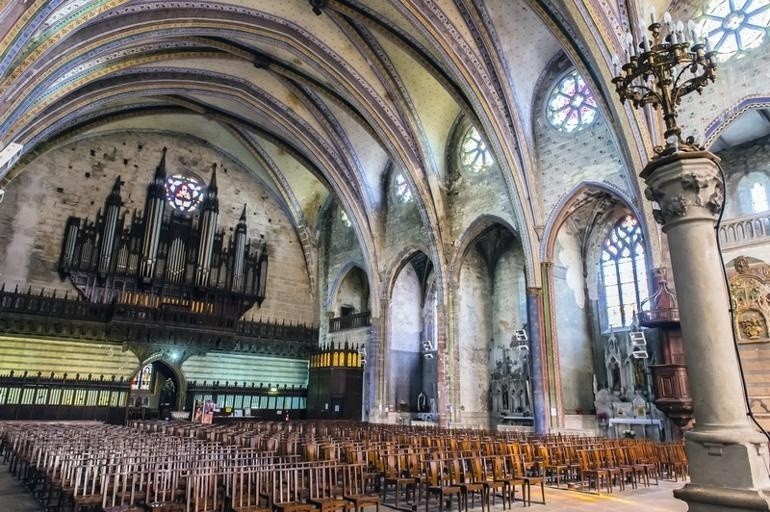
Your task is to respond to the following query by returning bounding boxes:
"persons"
[194,407,203,422]
[142,366,151,386]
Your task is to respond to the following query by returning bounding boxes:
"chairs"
[1,420,687,512]
